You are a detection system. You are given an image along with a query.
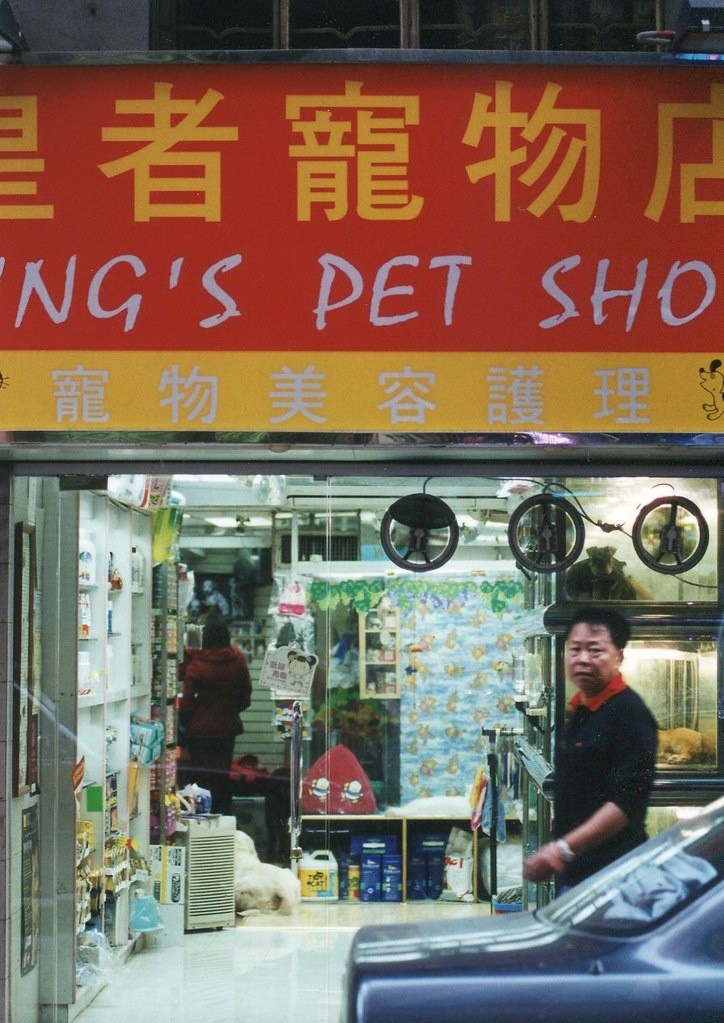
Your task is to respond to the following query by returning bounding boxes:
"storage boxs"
[350,835,402,902]
[407,832,446,900]
[144,905,183,948]
[151,845,186,905]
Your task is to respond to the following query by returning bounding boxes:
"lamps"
[286,486,508,511]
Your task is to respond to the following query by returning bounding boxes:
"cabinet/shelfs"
[152,561,179,846]
[358,606,402,699]
[36,491,154,1023]
[517,475,723,910]
[297,813,524,903]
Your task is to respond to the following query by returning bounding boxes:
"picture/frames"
[15,521,38,798]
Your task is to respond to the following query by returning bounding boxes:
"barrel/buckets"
[298,849,339,902]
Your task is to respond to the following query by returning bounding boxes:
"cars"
[340,798,724,1023]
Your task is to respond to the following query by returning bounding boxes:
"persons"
[523,608,659,900]
[180,618,251,815]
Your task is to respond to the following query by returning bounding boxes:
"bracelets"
[557,840,578,866]
